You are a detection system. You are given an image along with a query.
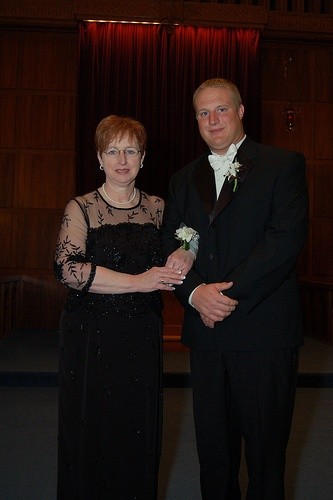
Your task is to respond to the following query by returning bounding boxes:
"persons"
[158,77,308,500]
[53,114,197,500]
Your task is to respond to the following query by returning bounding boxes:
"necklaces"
[102,182,136,204]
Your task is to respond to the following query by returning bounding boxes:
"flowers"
[218,158,249,193]
[175,222,199,254]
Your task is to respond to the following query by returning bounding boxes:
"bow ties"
[208,143,238,171]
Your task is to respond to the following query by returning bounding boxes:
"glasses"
[103,147,142,158]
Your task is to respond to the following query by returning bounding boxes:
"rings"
[161,277,165,284]
